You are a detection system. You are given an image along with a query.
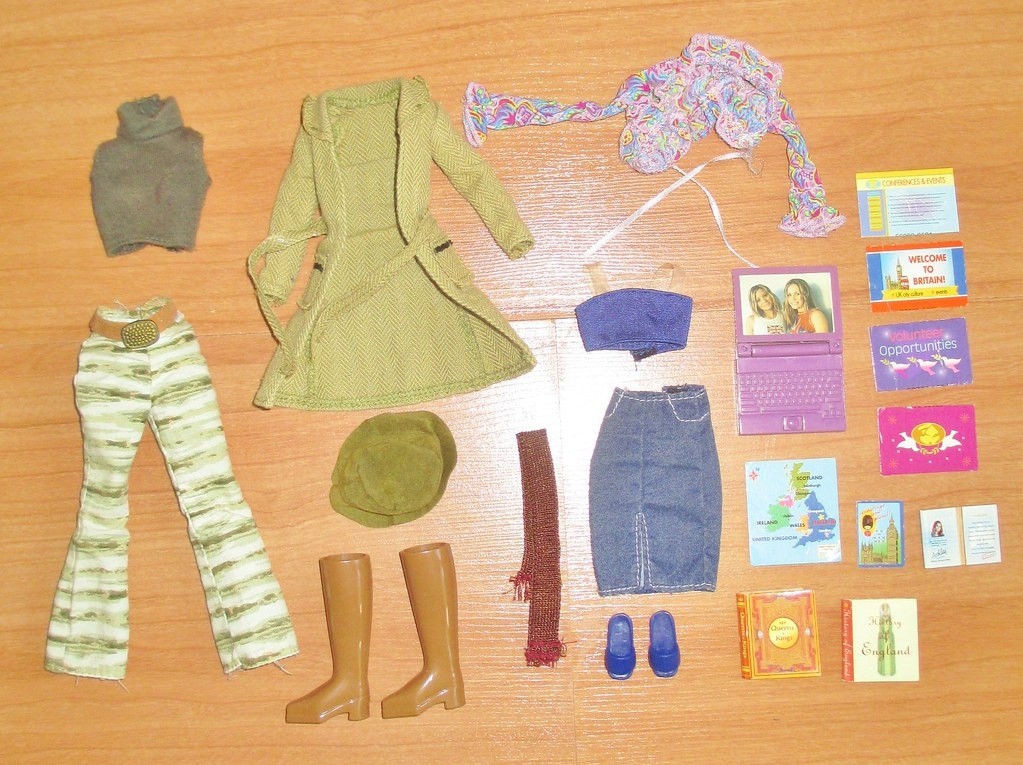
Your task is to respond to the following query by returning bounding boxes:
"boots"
[285,554,372,724]
[381,544,465,719]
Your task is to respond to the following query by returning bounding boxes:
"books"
[737,588,821,680]
[919,508,962,569]
[841,598,920,682]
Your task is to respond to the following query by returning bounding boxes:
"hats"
[330,411,457,528]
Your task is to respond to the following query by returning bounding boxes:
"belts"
[88,302,177,349]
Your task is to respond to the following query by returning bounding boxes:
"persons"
[784,279,829,334]
[931,522,945,537]
[746,284,786,335]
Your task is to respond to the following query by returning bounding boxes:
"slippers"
[601,613,636,680]
[649,610,680,678]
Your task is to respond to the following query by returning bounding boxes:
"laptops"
[732,265,847,435]
